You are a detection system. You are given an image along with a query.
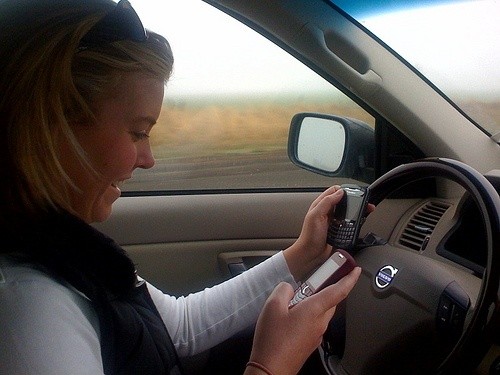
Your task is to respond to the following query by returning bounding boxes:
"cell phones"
[287,249,358,309]
[326,183,369,249]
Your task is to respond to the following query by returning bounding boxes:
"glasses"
[78,0,148,52]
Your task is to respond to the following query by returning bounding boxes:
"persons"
[2,0,363,375]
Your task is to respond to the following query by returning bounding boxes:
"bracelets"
[244,361,274,375]
